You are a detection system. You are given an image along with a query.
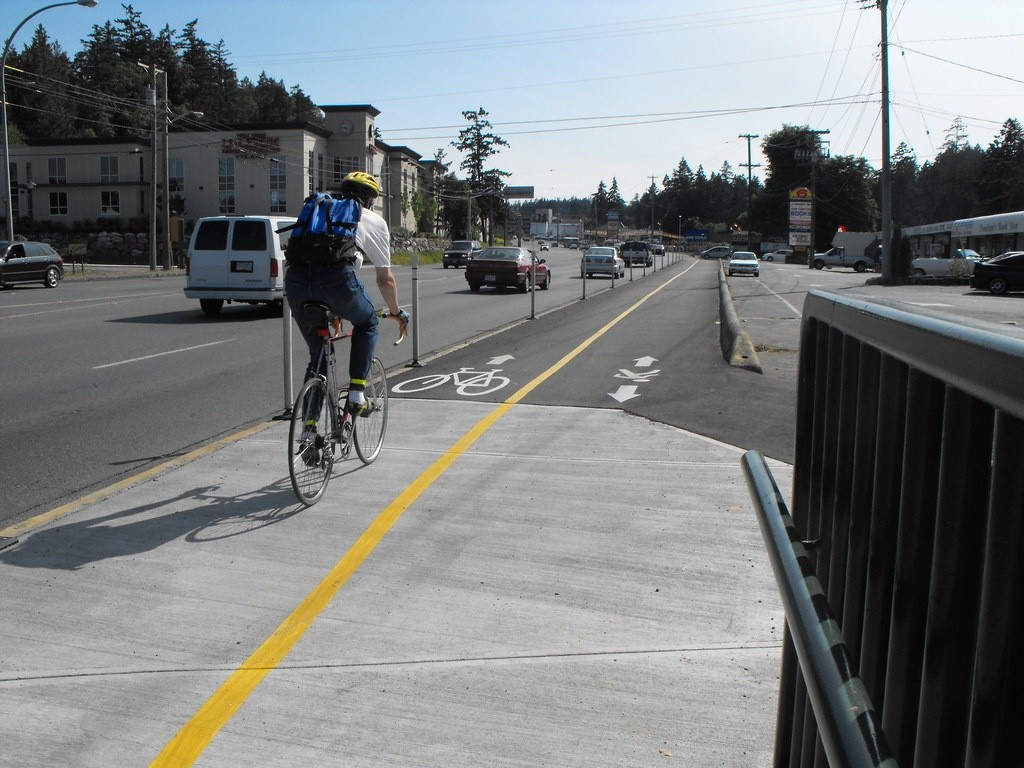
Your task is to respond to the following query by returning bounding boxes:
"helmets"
[341,172,380,199]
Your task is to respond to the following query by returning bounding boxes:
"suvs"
[443,240,483,269]
[620,241,653,267]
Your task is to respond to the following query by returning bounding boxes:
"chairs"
[735,256,740,258]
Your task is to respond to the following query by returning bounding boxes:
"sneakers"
[347,394,375,417]
[301,431,320,468]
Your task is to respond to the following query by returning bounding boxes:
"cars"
[700,246,732,260]
[580,247,625,279]
[649,244,665,257]
[729,251,761,277]
[569,239,626,251]
[0,241,64,290]
[465,246,551,294]
[761,249,793,262]
[512,235,563,251]
[911,249,990,277]
[970,251,1024,295]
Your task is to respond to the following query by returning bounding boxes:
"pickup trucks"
[808,247,875,273]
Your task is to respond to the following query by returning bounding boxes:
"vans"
[182,215,298,316]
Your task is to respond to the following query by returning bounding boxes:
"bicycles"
[288,301,409,507]
[0,0,99,241]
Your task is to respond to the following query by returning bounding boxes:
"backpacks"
[274,192,364,272]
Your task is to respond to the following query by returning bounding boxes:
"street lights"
[150,111,204,270]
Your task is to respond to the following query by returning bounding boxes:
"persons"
[284,171,411,466]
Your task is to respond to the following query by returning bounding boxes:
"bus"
[563,237,579,248]
